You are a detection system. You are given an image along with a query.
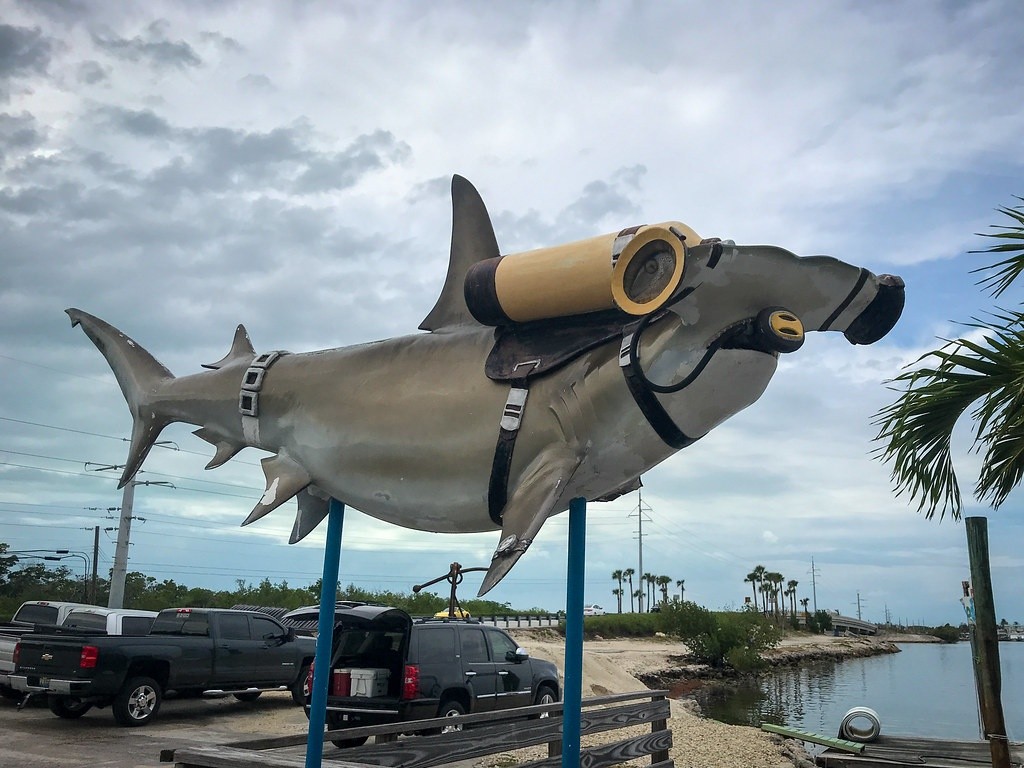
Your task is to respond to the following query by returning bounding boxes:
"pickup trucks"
[0,601,109,703]
[5,606,323,728]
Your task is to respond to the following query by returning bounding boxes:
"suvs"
[284,602,560,748]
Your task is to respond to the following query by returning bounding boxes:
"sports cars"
[433,608,471,622]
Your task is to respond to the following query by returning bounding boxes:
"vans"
[59,607,156,638]
